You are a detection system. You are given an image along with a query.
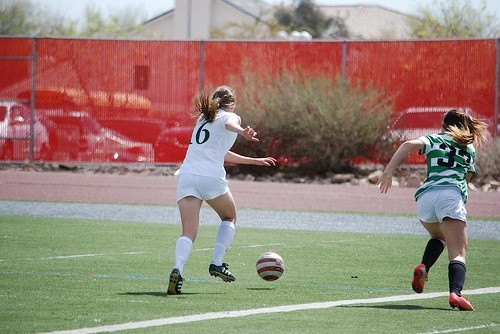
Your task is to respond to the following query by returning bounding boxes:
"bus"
[17,88,154,146]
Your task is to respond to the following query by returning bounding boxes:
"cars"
[1,100,49,163]
[101,127,149,162]
[375,106,490,164]
[41,108,104,163]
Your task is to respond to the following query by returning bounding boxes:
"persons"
[13,107,22,116]
[376,109,488,311]
[168,85,278,295]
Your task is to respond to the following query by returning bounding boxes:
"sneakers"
[209,263,236,282]
[448,292,475,311]
[167,269,185,295]
[412,264,429,293]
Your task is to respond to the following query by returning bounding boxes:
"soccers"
[256,252,285,281]
